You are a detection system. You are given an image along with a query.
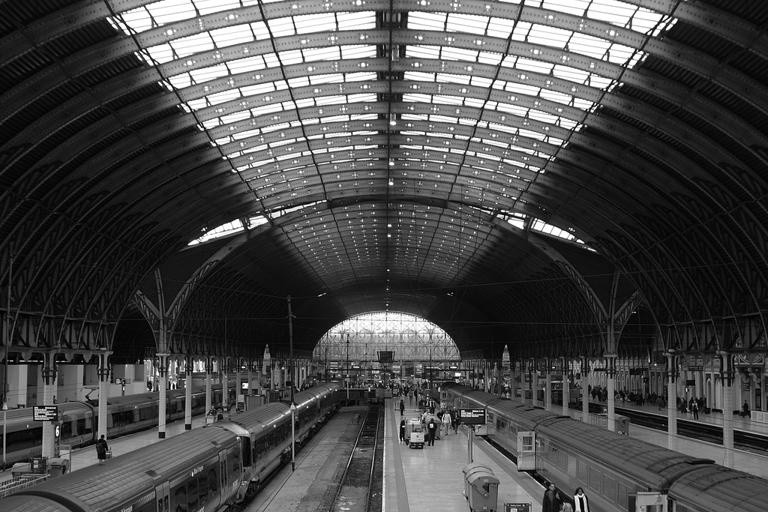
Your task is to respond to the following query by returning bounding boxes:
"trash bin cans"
[425,415,442,440]
[462,463,500,511]
[615,414,631,435]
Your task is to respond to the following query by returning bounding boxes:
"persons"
[573,487,590,512]
[207,405,217,421]
[741,399,751,419]
[95,433,109,463]
[542,483,560,512]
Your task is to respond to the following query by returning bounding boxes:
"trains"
[440,379,768,510]
